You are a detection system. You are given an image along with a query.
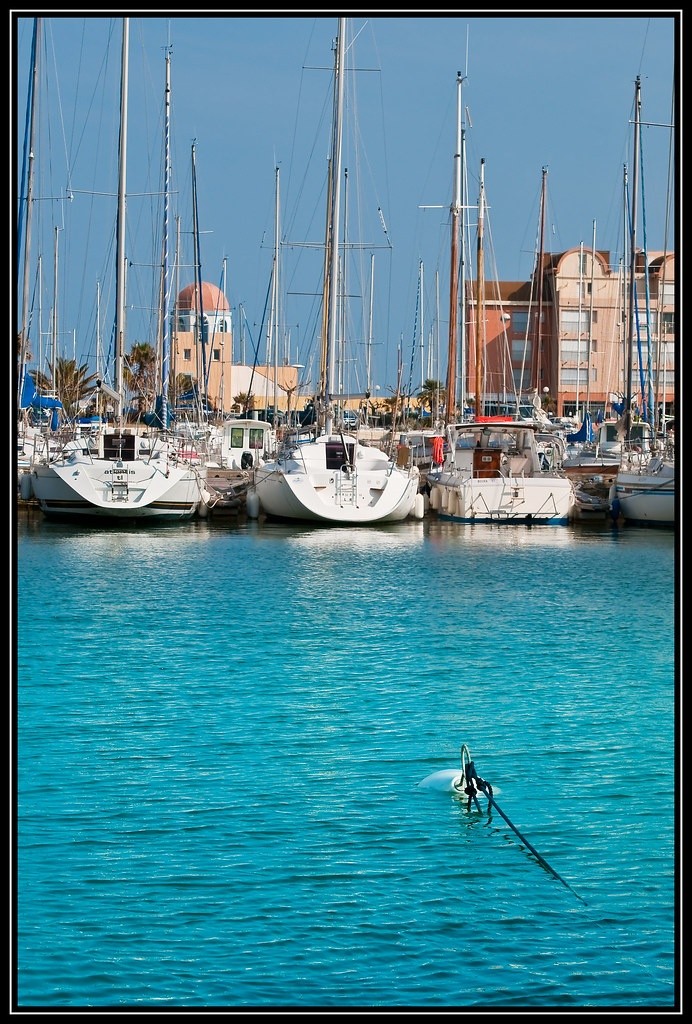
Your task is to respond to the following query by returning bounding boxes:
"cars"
[548,416,576,428]
[238,407,300,427]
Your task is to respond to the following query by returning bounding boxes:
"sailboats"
[11,12,684,532]
